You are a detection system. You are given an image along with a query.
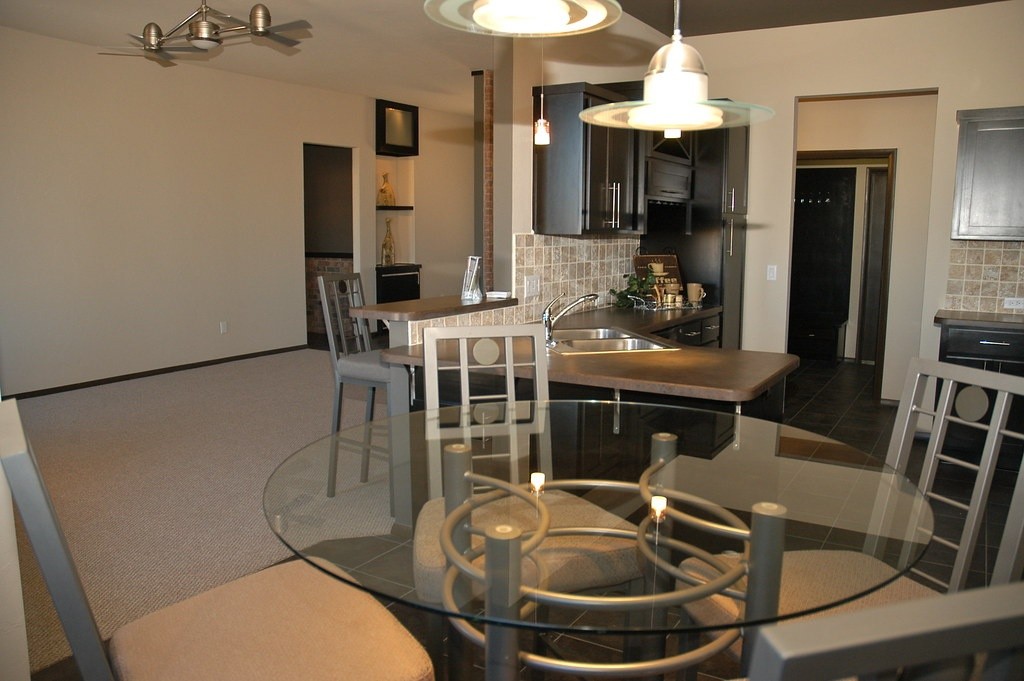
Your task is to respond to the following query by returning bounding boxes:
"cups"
[687,283,704,302]
[660,284,683,302]
[647,263,664,273]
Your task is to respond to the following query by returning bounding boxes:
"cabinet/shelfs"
[934,310,1024,478]
[375,262,422,331]
[530,83,747,473]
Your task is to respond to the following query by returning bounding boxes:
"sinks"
[550,327,631,341]
[558,338,665,352]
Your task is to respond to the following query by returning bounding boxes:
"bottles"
[381,217,395,267]
[376,172,396,206]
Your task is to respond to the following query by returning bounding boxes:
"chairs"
[667,356,1023,681]
[0,399,440,681]
[317,273,392,497]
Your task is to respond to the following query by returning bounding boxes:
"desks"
[263,401,938,681]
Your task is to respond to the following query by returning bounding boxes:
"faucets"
[542,292,598,347]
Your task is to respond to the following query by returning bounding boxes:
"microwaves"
[646,159,692,203]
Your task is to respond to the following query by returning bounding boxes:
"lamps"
[424,0,621,144]
[579,1,775,140]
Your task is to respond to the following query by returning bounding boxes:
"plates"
[652,272,669,277]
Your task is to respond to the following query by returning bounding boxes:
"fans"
[97,0,312,67]
